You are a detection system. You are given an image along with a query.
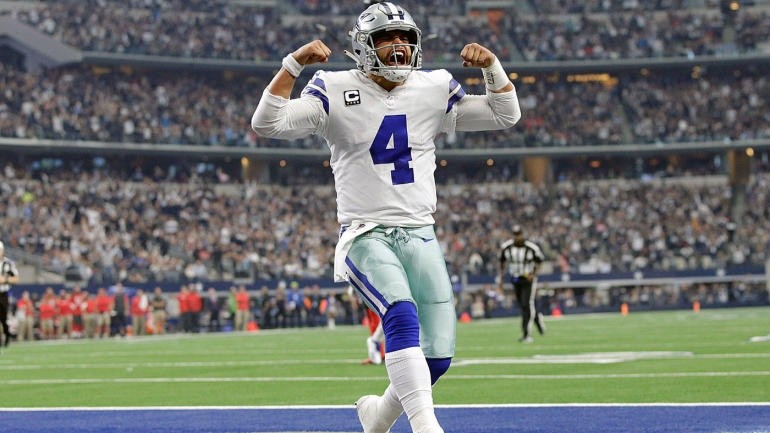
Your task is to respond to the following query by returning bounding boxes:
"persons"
[363,296,385,365]
[497,224,546,344]
[0,242,19,347]
[0,0,369,343]
[251,0,521,433]
[399,0,770,318]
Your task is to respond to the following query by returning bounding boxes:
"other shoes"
[366,337,382,365]
[354,395,390,433]
[517,335,533,344]
[534,312,546,334]
[4,333,11,346]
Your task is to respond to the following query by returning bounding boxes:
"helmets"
[344,1,422,82]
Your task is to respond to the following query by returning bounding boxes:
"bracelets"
[281,53,306,77]
